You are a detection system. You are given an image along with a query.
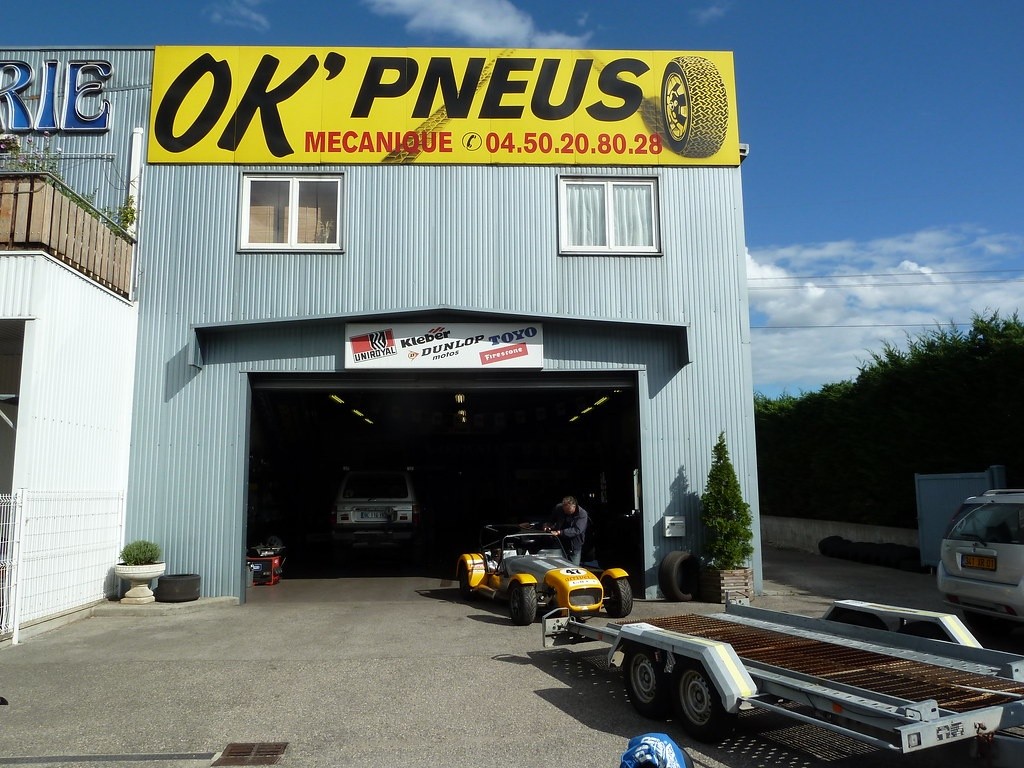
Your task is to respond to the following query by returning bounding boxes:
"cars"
[456,530,635,627]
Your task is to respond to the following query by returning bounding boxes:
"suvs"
[938,489,1023,622]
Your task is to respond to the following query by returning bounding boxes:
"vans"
[330,471,421,548]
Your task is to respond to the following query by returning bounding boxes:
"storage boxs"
[250,181,337,243]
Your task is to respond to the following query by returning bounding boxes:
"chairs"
[492,548,521,563]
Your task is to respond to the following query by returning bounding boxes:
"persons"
[543,495,588,566]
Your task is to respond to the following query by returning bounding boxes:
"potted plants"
[699,432,754,604]
[116,540,165,604]
[318,220,335,243]
